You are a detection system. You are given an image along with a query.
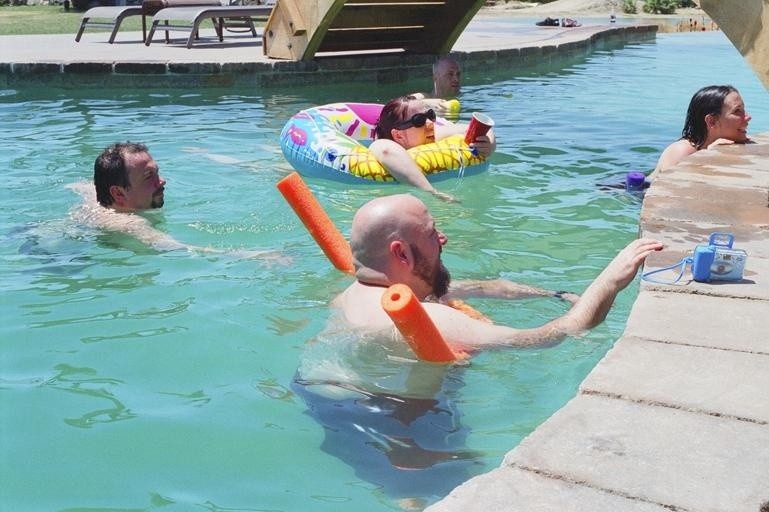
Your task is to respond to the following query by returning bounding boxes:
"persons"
[293,189,668,510]
[432,57,462,94]
[22,137,292,271]
[642,83,753,180]
[366,92,497,204]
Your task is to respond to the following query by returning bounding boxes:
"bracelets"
[554,289,576,302]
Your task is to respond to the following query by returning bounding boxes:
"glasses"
[393,108,437,129]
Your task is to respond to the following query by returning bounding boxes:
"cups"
[464,112,495,149]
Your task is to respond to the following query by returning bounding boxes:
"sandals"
[535,16,582,28]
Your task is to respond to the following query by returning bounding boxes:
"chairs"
[75,1,275,50]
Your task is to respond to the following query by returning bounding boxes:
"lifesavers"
[279,102,490,185]
